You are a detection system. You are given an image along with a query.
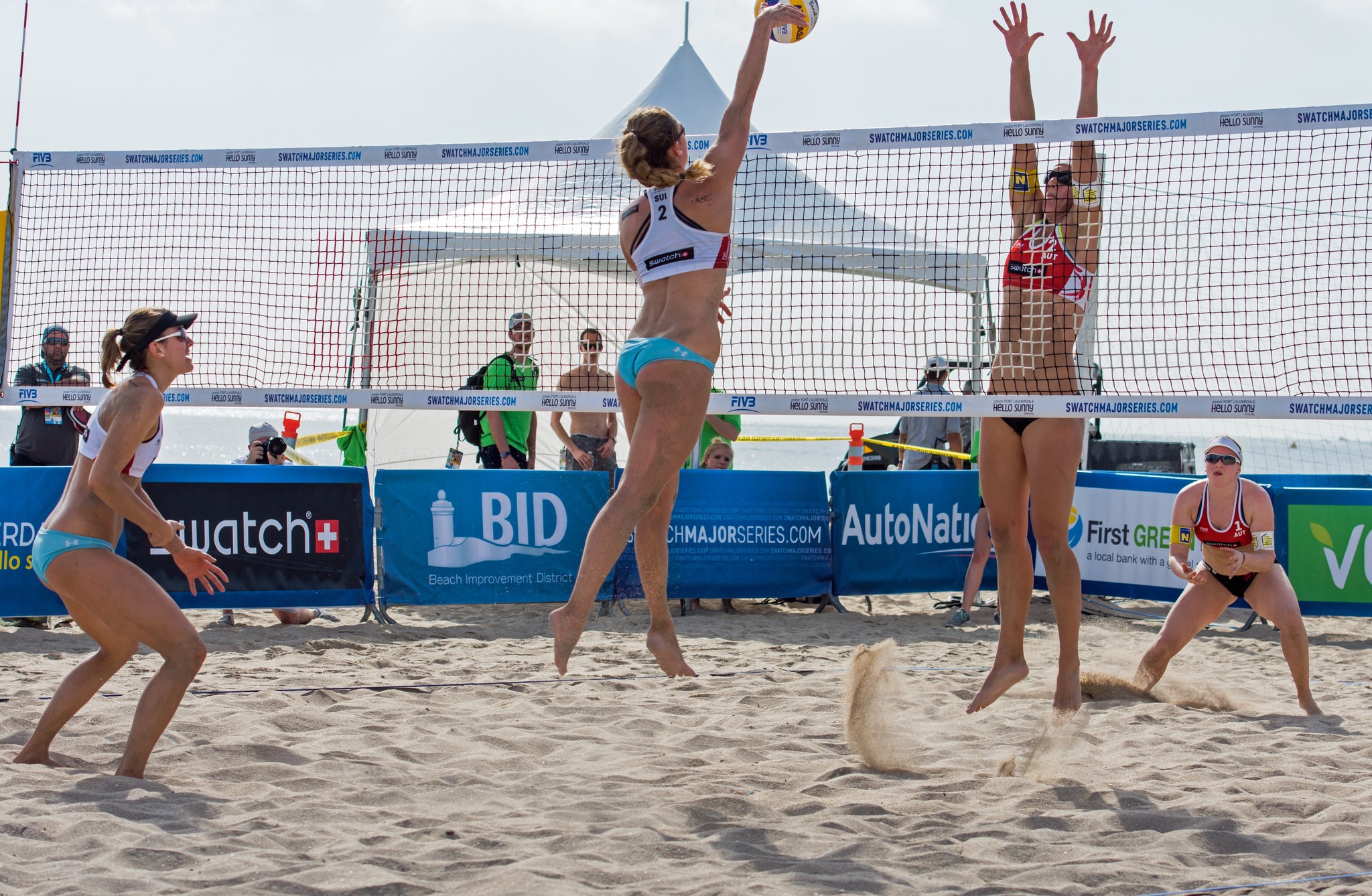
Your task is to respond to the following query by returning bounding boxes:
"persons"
[897,355,963,470]
[549,2,809,680]
[1132,437,1325,716]
[946,427,1000,628]
[12,324,91,466]
[12,308,229,781]
[550,328,619,498]
[679,387,742,614]
[966,1,1117,726]
[216,423,342,627]
[479,313,540,469]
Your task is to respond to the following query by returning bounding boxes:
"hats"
[1203,436,1243,466]
[508,313,534,329]
[248,421,279,444]
[928,356,947,371]
[116,309,198,372]
[40,325,69,359]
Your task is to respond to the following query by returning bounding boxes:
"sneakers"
[216,613,235,626]
[945,606,971,627]
[991,612,1001,625]
[315,608,340,623]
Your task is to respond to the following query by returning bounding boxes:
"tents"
[338,2,986,469]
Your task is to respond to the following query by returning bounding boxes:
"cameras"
[256,437,287,464]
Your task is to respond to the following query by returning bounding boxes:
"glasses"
[1205,453,1240,465]
[154,328,187,342]
[43,337,69,346]
[1043,170,1072,186]
[581,343,602,351]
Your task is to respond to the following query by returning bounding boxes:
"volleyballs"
[754,0,819,44]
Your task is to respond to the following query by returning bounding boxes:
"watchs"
[609,436,617,443]
[500,451,511,459]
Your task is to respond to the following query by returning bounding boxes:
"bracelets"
[1238,551,1246,565]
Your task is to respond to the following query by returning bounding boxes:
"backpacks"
[453,354,539,447]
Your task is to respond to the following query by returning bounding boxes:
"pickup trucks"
[836,359,1196,475]
